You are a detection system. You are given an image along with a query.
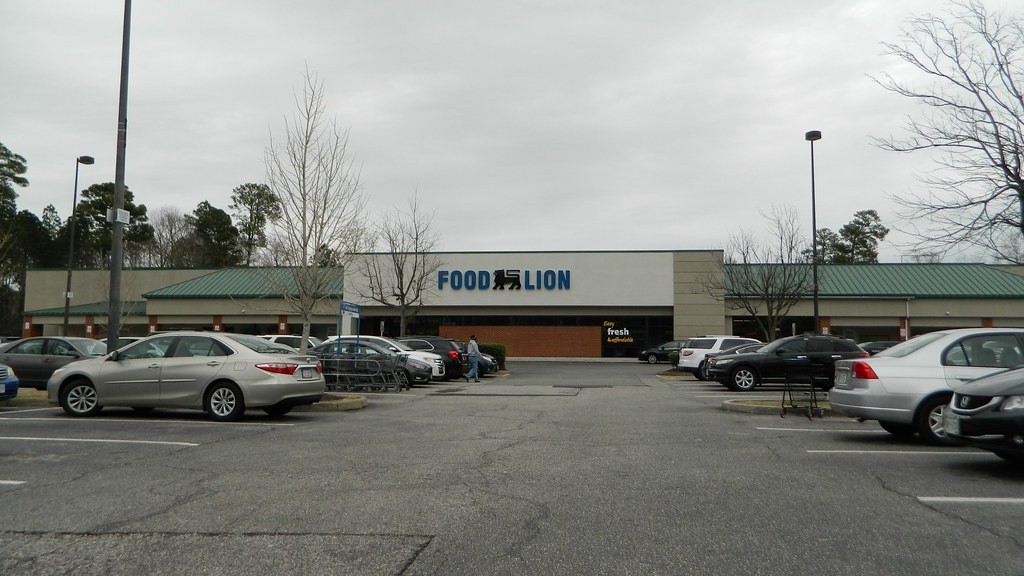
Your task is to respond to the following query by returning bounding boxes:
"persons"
[464,335,483,382]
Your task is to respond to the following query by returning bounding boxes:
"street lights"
[805,130,821,335]
[62,155,95,337]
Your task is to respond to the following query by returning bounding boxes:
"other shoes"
[475,381,480,383]
[466,377,468,383]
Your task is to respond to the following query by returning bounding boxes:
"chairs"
[973,348,999,367]
[996,347,1021,368]
[173,343,195,357]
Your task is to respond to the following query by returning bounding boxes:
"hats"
[471,335,477,340]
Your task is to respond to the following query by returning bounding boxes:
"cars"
[46,331,326,422]
[677,335,762,381]
[704,343,767,386]
[858,342,1021,366]
[708,335,870,392]
[942,368,1023,463]
[89,337,164,356]
[638,340,686,365]
[827,327,1024,447]
[0,337,107,390]
[258,336,499,387]
